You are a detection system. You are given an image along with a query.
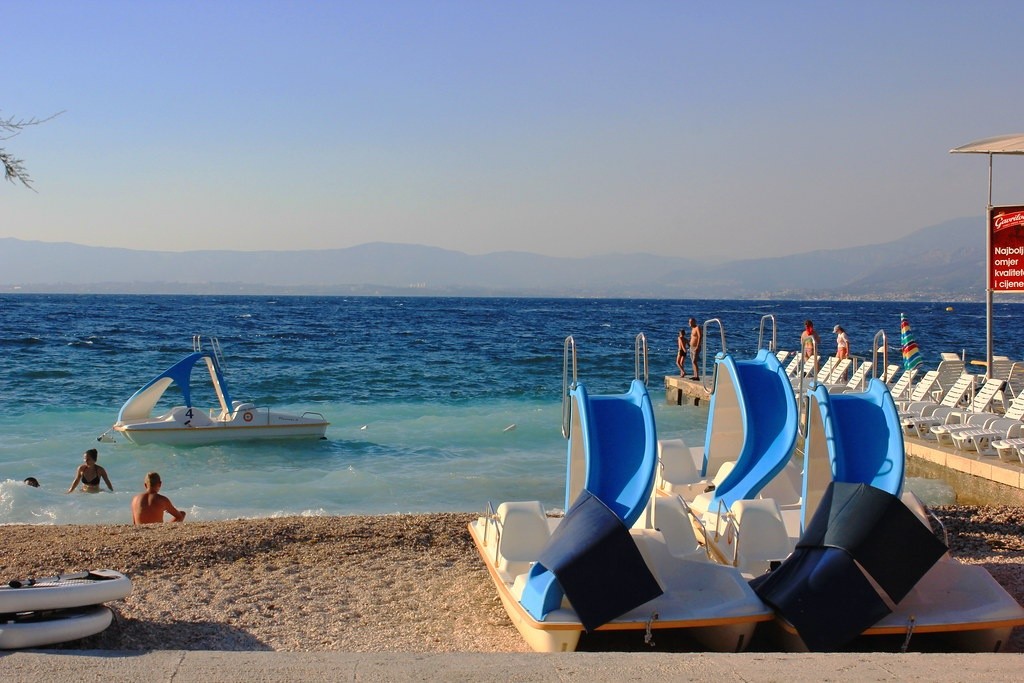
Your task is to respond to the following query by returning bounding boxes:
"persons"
[687,317,701,381]
[131,473,186,524]
[62,448,116,496]
[800,320,821,376]
[23,477,41,490]
[834,324,850,382]
[677,330,690,378]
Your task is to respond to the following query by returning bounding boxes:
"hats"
[832,325,841,333]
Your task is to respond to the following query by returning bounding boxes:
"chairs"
[785,353,804,377]
[892,370,941,405]
[990,362,1024,413]
[890,368,918,397]
[900,379,1005,438]
[777,350,789,363]
[790,355,822,388]
[916,361,965,398]
[827,361,874,394]
[496,501,789,582]
[941,352,981,404]
[991,438,1024,464]
[897,374,975,432]
[975,360,1015,393]
[843,365,900,395]
[658,438,709,501]
[929,389,1024,451]
[823,358,852,390]
[790,356,839,391]
[950,419,1024,456]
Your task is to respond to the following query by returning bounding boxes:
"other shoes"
[680,372,686,378]
[689,377,700,381]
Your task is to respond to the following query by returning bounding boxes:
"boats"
[468,313,1024,655]
[111,334,331,445]
